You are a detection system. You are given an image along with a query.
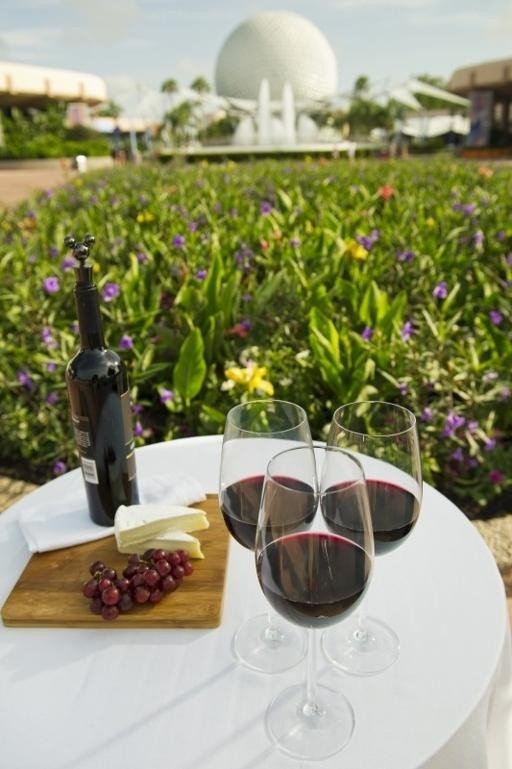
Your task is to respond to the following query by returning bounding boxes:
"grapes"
[83,548,194,621]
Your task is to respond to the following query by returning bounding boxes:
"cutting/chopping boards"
[2,492,230,628]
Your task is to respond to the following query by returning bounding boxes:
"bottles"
[62,236,140,528]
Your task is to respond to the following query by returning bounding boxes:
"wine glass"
[324,403,424,679]
[252,446,376,758]
[214,402,310,673]
